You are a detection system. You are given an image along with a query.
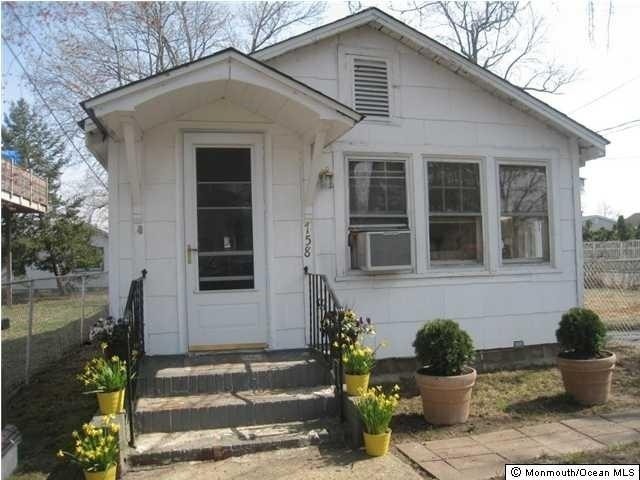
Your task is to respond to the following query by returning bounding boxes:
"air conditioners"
[356,229,416,274]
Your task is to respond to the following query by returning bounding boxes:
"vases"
[360,428,392,457]
[81,462,118,480]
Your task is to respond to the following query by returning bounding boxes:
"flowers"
[319,305,401,434]
[55,315,136,471]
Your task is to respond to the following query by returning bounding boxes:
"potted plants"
[556,307,617,408]
[412,319,478,427]
